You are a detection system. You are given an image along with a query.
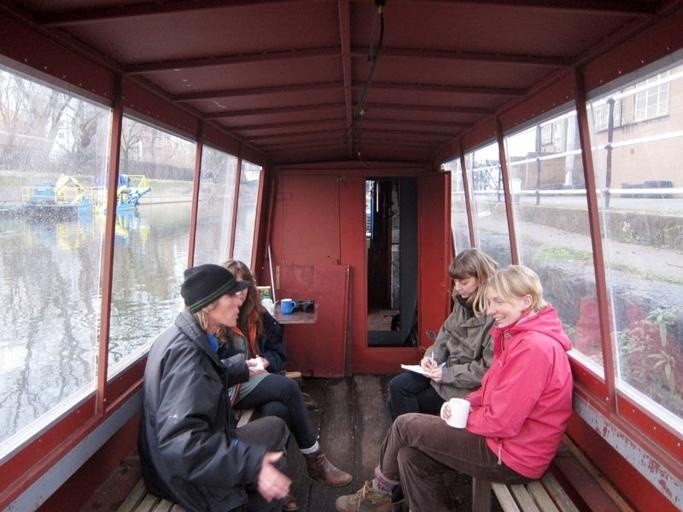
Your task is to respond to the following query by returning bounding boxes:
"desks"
[274,299,319,324]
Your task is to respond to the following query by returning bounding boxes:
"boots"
[281,493,299,511]
[335,481,392,511]
[302,447,353,488]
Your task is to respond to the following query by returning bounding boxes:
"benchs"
[472,432,631,511]
[119,403,254,512]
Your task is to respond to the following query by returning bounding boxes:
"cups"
[440,397,471,430]
[280,298,296,315]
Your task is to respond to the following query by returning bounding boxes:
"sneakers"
[392,486,404,504]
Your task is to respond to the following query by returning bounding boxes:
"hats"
[181,265,252,314]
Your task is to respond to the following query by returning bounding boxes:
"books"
[400,361,447,378]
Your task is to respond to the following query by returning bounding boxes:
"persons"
[388,248,501,423]
[334,264,573,512]
[136,263,292,512]
[221,260,354,489]
